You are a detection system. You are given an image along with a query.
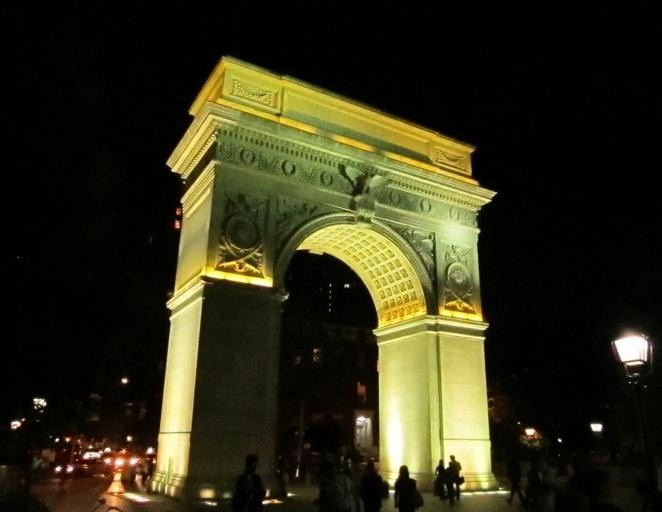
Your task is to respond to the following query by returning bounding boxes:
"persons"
[311,446,462,512]
[231,454,266,512]
[506,454,623,512]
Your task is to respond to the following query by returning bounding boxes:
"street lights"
[611,328,656,503]
[10,395,49,503]
[524,427,536,471]
[590,421,605,438]
